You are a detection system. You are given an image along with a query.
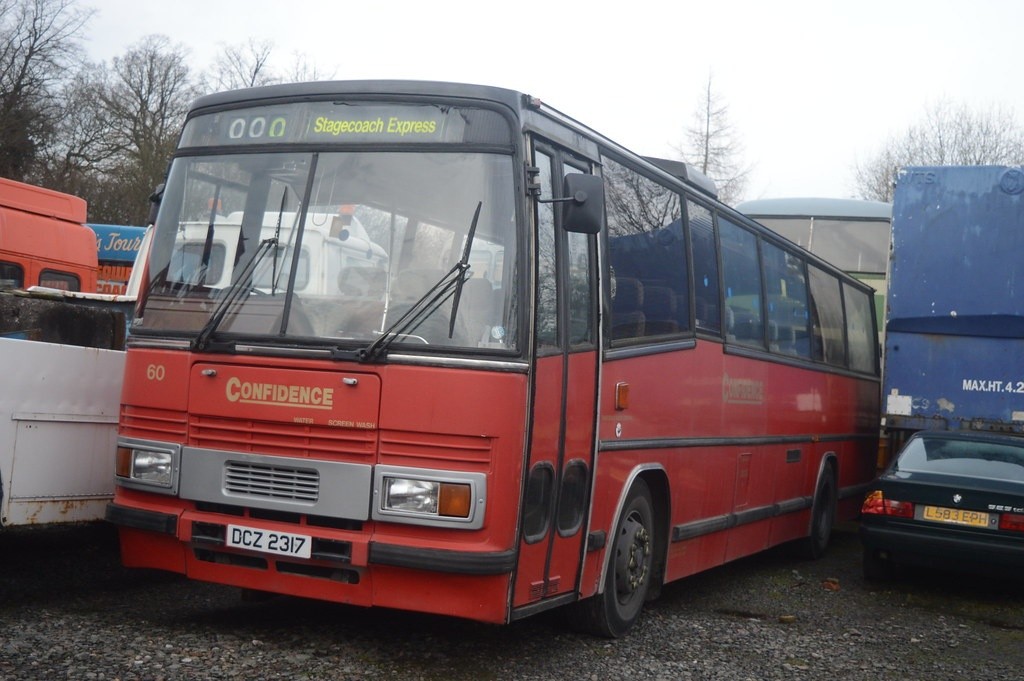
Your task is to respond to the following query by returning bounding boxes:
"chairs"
[332,263,503,346]
[605,275,811,354]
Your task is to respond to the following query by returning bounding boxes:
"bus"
[103,78,882,640]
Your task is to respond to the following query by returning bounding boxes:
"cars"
[862,429,1024,584]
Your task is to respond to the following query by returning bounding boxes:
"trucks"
[0,197,391,549]
